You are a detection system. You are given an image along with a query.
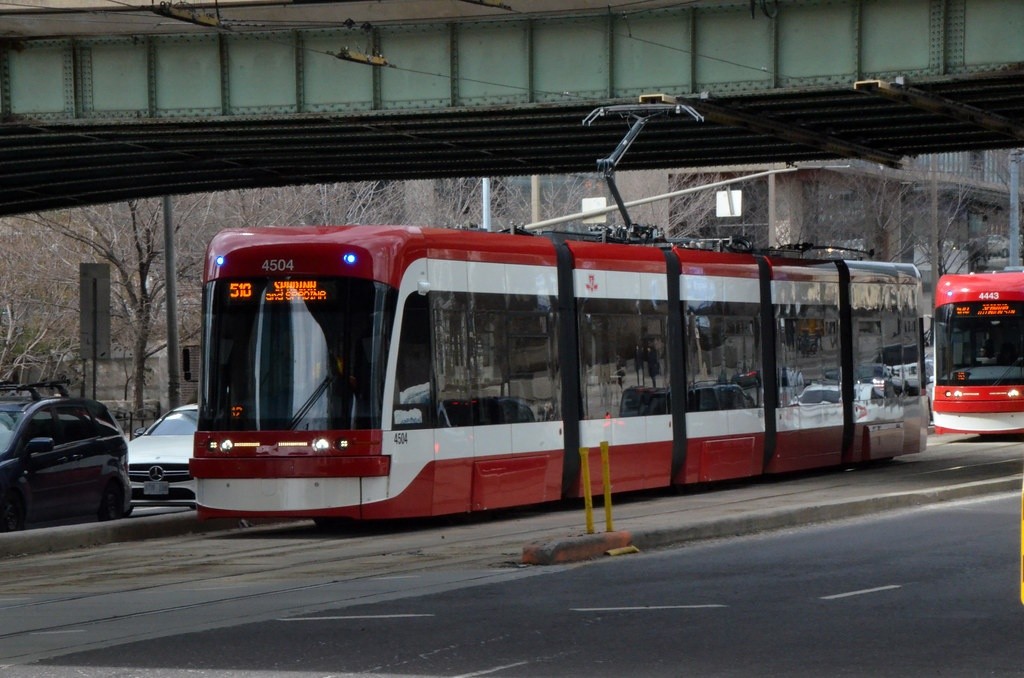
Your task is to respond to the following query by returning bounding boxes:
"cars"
[728,344,919,407]
[967,235,1010,257]
[925,358,933,422]
[822,238,866,259]
[127,403,198,514]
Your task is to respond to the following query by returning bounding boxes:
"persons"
[633,344,646,386]
[645,347,659,388]
[616,354,625,389]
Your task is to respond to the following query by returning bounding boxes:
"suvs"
[617,381,754,415]
[0,379,130,532]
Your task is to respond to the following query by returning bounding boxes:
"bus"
[181,105,929,531]
[923,274,1024,440]
